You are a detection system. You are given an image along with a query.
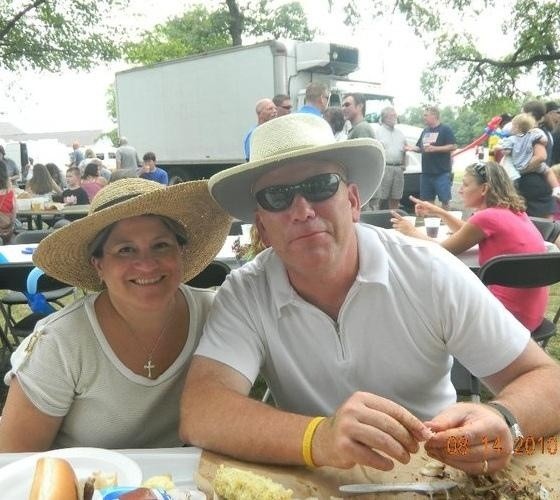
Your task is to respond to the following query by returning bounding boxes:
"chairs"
[0,192,560,398]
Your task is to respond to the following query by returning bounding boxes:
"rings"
[484,460,488,475]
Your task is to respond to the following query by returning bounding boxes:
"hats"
[499,113,516,125]
[209,114,386,225]
[545,101,560,113]
[32,178,234,291]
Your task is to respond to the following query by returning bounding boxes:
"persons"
[242,81,408,211]
[476,100,559,220]
[0,136,169,245]
[179,112,560,477]
[404,106,457,210]
[390,161,548,335]
[0,176,236,451]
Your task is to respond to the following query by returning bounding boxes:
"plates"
[0,446,143,500]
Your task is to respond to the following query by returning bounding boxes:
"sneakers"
[551,187,560,200]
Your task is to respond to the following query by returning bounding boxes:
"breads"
[28,456,78,500]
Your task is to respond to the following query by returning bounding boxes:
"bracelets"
[302,416,326,469]
[488,401,523,453]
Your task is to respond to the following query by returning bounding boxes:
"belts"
[386,164,404,165]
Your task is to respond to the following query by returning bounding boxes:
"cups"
[403,216,416,226]
[31,198,41,211]
[424,217,441,238]
[449,210,462,221]
[241,223,252,240]
[424,143,431,152]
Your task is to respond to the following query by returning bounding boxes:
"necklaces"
[117,308,170,378]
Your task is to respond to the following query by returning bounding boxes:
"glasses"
[255,172,348,212]
[470,163,486,183]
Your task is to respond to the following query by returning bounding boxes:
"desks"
[0,441,559,499]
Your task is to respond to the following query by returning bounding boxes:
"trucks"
[114,41,454,210]
